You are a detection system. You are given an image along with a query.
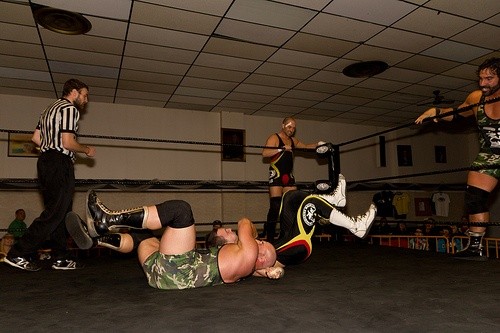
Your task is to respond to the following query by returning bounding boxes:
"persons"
[414,56,500,257]
[6,208,38,261]
[263,117,332,243]
[206,173,377,266]
[4,77,97,272]
[213,220,223,231]
[64,185,277,292]
[372,212,469,255]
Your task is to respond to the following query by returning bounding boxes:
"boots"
[85,187,148,238]
[318,173,347,208]
[452,231,488,257]
[65,210,123,252]
[328,203,377,240]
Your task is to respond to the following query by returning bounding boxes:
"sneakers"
[51,257,87,270]
[3,253,45,271]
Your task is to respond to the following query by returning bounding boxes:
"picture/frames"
[221,128,246,162]
[8,132,39,157]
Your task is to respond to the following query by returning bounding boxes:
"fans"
[416,90,455,106]
